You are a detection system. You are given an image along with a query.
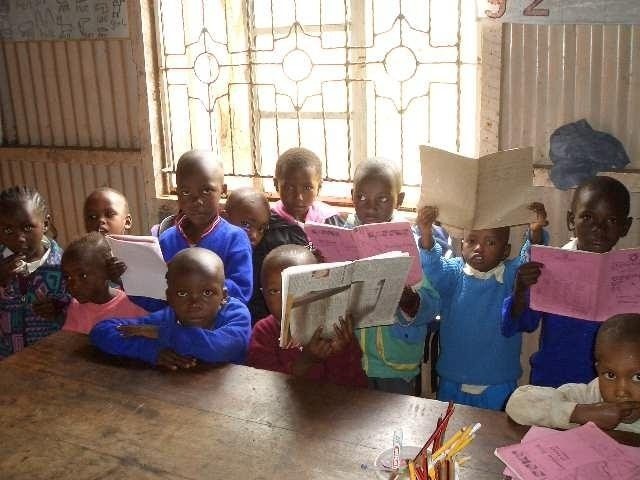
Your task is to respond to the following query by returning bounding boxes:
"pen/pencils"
[360,463,399,472]
[407,400,482,480]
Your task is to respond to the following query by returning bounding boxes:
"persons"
[88,246,253,372]
[252,143,345,327]
[501,175,640,386]
[500,312,639,434]
[1,183,74,362]
[413,200,553,413]
[333,155,442,399]
[59,231,153,334]
[130,147,256,314]
[82,184,168,314]
[223,186,270,251]
[246,243,363,385]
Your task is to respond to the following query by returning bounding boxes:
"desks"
[1,323,639,479]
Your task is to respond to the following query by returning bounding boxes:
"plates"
[374,444,460,479]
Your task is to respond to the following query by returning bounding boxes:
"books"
[103,233,169,303]
[526,240,639,323]
[300,218,424,291]
[417,144,542,232]
[488,422,639,480]
[278,250,412,350]
